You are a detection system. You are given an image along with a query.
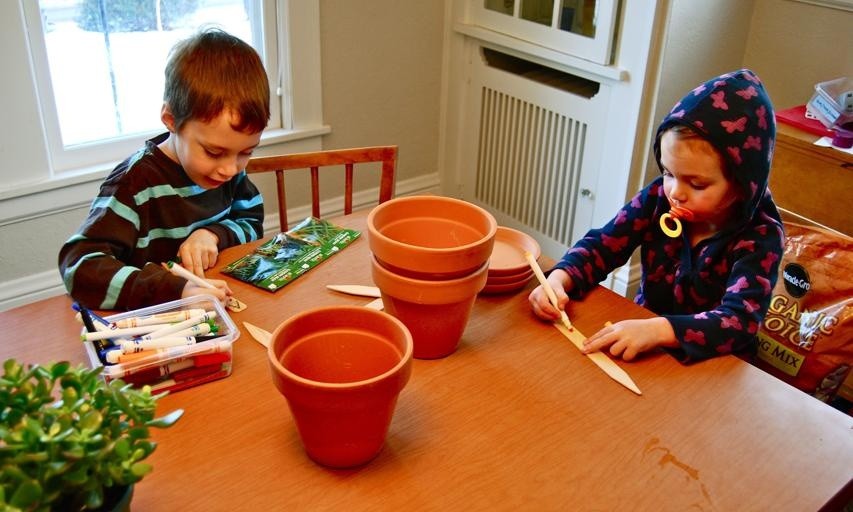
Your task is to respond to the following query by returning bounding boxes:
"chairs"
[754,221,853,406]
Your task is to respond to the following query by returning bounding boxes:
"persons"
[54,25,273,318]
[526,66,787,369]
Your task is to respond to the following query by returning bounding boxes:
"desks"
[0,203,853,510]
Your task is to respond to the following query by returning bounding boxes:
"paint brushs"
[164,264,235,306]
[525,253,578,335]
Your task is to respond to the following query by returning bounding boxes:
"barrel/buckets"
[372,257,487,358]
[369,196,495,271]
[271,309,413,470]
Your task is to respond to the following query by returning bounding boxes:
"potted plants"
[0,357,185,512]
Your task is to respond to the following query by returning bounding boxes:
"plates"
[481,227,541,300]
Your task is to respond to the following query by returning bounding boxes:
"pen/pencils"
[74,303,244,390]
